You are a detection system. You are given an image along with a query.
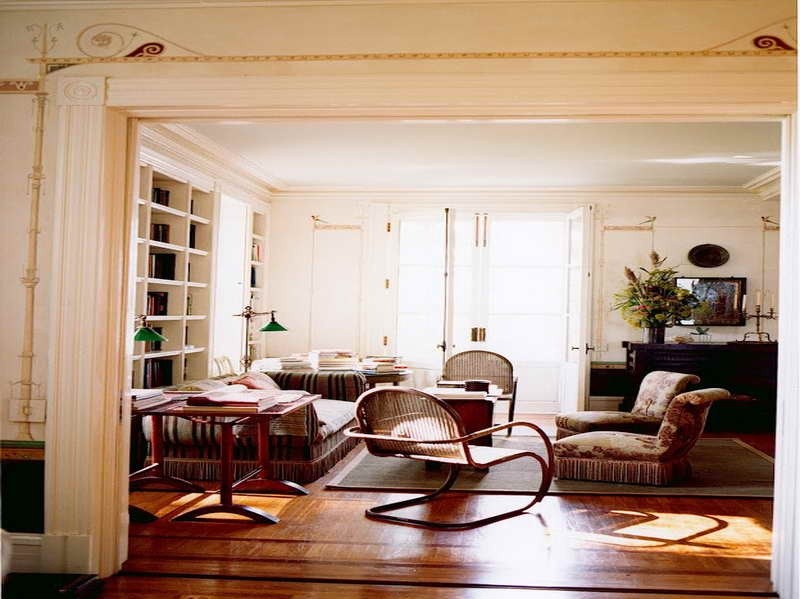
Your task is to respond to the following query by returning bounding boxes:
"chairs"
[437,350,518,436]
[343,385,554,528]
[552,370,731,486]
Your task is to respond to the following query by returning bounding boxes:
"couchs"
[141,371,367,481]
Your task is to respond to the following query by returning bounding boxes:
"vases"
[648,323,664,344]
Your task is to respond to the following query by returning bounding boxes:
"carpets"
[326,434,774,497]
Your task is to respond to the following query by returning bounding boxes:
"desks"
[282,368,409,389]
[129,394,323,524]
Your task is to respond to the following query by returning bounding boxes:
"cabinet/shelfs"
[131,164,216,388]
[622,340,778,434]
[244,208,268,359]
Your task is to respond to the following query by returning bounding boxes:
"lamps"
[233,306,288,372]
[134,313,170,342]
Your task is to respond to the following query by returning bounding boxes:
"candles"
[770,294,775,306]
[742,294,747,311]
[756,292,760,305]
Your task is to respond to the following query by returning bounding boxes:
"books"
[142,189,195,387]
[251,243,263,288]
[279,349,409,375]
[131,388,279,413]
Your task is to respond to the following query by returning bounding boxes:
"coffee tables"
[425,389,503,472]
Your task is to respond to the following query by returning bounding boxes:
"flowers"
[607,250,714,328]
